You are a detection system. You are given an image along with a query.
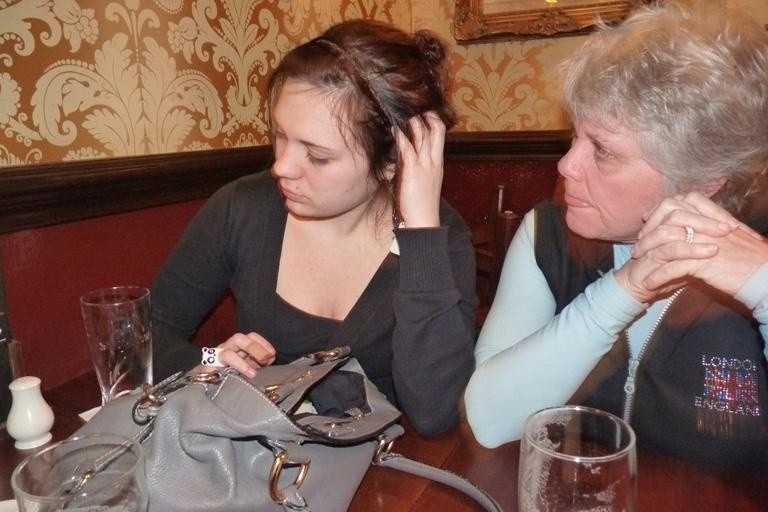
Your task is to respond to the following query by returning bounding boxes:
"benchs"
[0,130,768,394]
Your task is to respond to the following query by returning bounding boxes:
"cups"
[11,434,144,512]
[518,405,637,511]
[80,286,153,408]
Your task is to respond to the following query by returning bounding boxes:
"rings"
[685,227,696,245]
[199,346,227,367]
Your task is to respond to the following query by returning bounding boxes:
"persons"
[462,0,768,473]
[114,16,482,441]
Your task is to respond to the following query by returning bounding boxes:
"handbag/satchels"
[48,343,405,512]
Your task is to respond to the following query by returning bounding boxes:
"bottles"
[6,376,55,449]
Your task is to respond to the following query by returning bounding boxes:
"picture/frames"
[453,0,652,43]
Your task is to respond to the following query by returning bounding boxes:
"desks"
[1,360,768,512]
